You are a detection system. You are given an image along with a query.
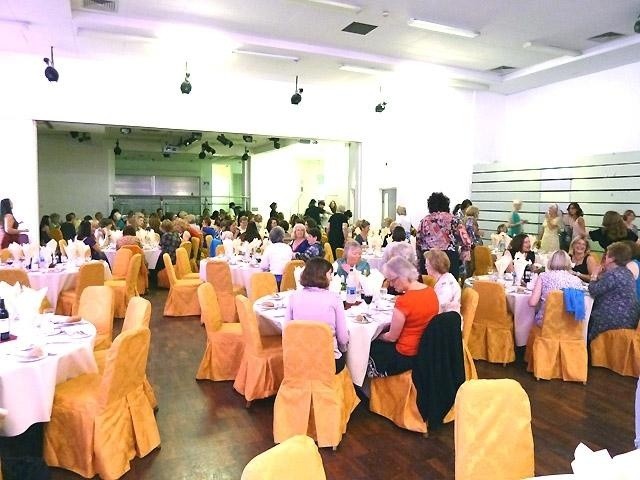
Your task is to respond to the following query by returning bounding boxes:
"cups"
[39,261,49,273]
[380,288,388,301]
[42,308,58,337]
[231,256,238,265]
[54,264,64,273]
[487,267,495,279]
[288,288,294,297]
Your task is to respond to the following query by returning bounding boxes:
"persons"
[174,218,201,276]
[396,205,411,243]
[269,202,278,217]
[379,218,391,238]
[284,257,350,375]
[245,211,263,222]
[134,212,144,230]
[50,213,61,231]
[148,220,181,288]
[585,241,640,342]
[380,222,400,248]
[304,199,324,225]
[507,199,528,240]
[289,215,307,233]
[354,219,371,245]
[218,220,240,241]
[565,202,586,239]
[276,212,285,223]
[210,210,220,226]
[351,220,363,237]
[570,236,599,280]
[60,214,77,245]
[157,208,165,222]
[260,226,297,282]
[538,201,563,253]
[345,210,352,219]
[365,256,442,378]
[165,211,176,221]
[414,192,472,282]
[177,211,201,228]
[96,219,117,246]
[198,208,211,227]
[460,207,483,280]
[201,217,215,248]
[0,198,30,250]
[456,200,471,215]
[264,218,278,240]
[626,240,640,302]
[116,226,149,275]
[73,220,113,276]
[220,209,231,223]
[382,227,416,272]
[278,221,291,244]
[107,209,124,231]
[238,216,249,233]
[40,215,52,247]
[497,225,508,236]
[424,249,464,336]
[574,202,640,265]
[240,221,263,246]
[229,202,245,227]
[330,201,336,210]
[326,204,348,260]
[318,201,327,218]
[146,213,164,237]
[527,251,585,329]
[623,210,640,236]
[125,211,133,221]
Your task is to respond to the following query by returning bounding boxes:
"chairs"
[0,269,50,314]
[279,260,305,292]
[103,248,132,281]
[589,320,640,378]
[524,291,589,384]
[188,237,199,273]
[56,263,105,316]
[156,241,191,287]
[250,272,278,300]
[162,254,203,316]
[324,243,334,263]
[454,378,534,478]
[232,294,284,408]
[463,289,480,344]
[176,247,201,279]
[467,280,515,367]
[75,286,114,352]
[121,245,148,295]
[0,248,12,260]
[196,282,281,381]
[105,254,142,318]
[369,312,479,438]
[43,326,161,479]
[472,245,493,277]
[239,433,325,479]
[96,296,158,410]
[205,235,216,257]
[273,319,361,450]
[200,261,246,325]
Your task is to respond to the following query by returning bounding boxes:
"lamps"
[180,62,191,94]
[43,47,58,81]
[290,76,303,105]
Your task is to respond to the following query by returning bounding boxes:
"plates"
[61,325,94,339]
[260,302,278,310]
[354,314,373,323]
[270,292,282,302]
[371,301,394,310]
[47,316,85,326]
[15,348,45,362]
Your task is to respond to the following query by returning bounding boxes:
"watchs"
[576,271,583,280]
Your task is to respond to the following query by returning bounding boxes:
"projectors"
[161,145,179,154]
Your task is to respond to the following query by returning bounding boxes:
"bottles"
[346,269,357,303]
[0,298,10,342]
[524,255,531,283]
